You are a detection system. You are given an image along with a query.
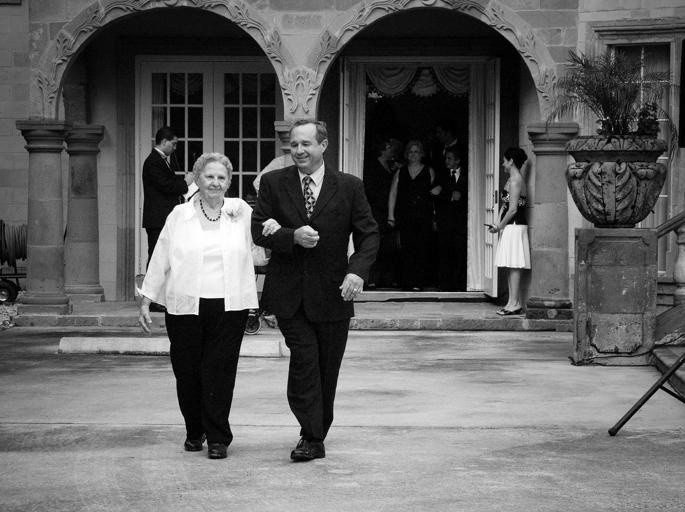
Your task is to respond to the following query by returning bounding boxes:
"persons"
[385,140,435,289]
[375,137,402,179]
[487,147,531,317]
[249,120,380,460]
[137,152,283,460]
[432,121,466,152]
[431,145,467,289]
[142,126,194,313]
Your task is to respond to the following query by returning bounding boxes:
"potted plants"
[547,45,684,227]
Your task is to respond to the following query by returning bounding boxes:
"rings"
[269,227,274,231]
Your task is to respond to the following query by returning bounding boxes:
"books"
[182,181,199,202]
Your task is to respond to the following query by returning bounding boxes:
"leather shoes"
[291,434,325,461]
[208,443,227,458]
[184,434,204,451]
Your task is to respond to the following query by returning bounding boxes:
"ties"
[303,175,316,220]
[452,170,456,182]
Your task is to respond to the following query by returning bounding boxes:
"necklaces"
[199,197,225,222]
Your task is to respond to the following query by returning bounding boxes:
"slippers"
[496,306,522,315]
